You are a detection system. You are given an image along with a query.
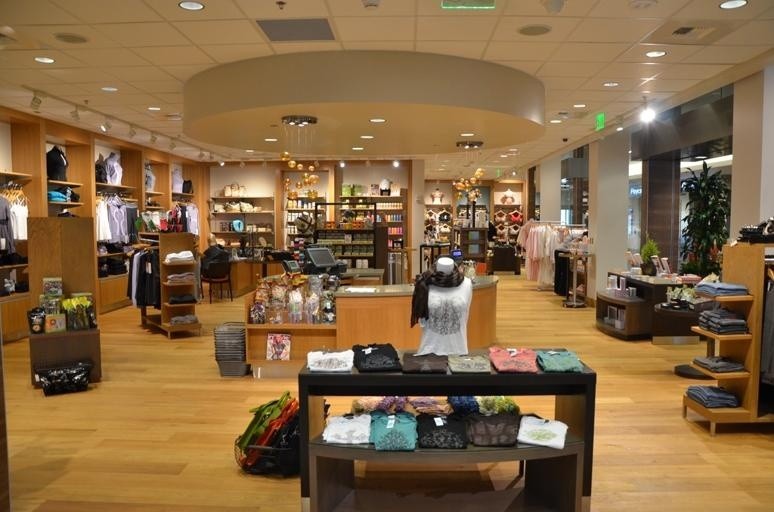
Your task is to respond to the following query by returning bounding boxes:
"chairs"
[200,261,233,303]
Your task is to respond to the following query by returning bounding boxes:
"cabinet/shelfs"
[209,192,279,261]
[452,226,487,263]
[595,268,700,341]
[681,239,774,436]
[136,231,201,339]
[284,198,329,254]
[297,348,596,511]
[145,146,171,231]
[44,118,96,219]
[425,204,452,260]
[333,188,425,284]
[28,218,101,389]
[313,202,389,283]
[209,262,262,300]
[455,205,494,227]
[94,133,145,315]
[555,247,593,308]
[493,204,528,263]
[264,262,285,278]
[171,155,203,255]
[244,284,336,379]
[0,107,41,344]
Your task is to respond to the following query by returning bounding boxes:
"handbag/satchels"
[34,362,91,396]
[236,391,330,478]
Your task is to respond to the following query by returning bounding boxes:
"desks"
[420,242,450,273]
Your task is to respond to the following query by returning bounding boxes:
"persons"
[47,145,68,181]
[417,257,472,356]
[431,187,444,204]
[271,334,288,360]
[104,152,123,185]
[172,169,184,193]
[145,164,156,191]
[503,188,514,204]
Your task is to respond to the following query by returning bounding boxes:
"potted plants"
[638,231,660,276]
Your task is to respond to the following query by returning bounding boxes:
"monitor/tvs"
[307,248,336,272]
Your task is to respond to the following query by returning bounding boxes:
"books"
[267,333,290,360]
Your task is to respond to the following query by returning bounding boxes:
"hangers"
[427,209,436,214]
[508,208,523,216]
[495,223,507,228]
[509,223,522,229]
[96,191,125,205]
[0,182,31,206]
[439,223,451,229]
[439,208,451,215]
[494,208,507,215]
[426,223,435,227]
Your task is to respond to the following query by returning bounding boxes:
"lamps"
[30,92,41,114]
[100,116,112,132]
[70,105,81,121]
[129,123,137,136]
[452,141,485,202]
[167,137,177,150]
[149,131,156,144]
[217,158,225,166]
[280,115,319,202]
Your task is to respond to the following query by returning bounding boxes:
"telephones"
[282,260,301,272]
